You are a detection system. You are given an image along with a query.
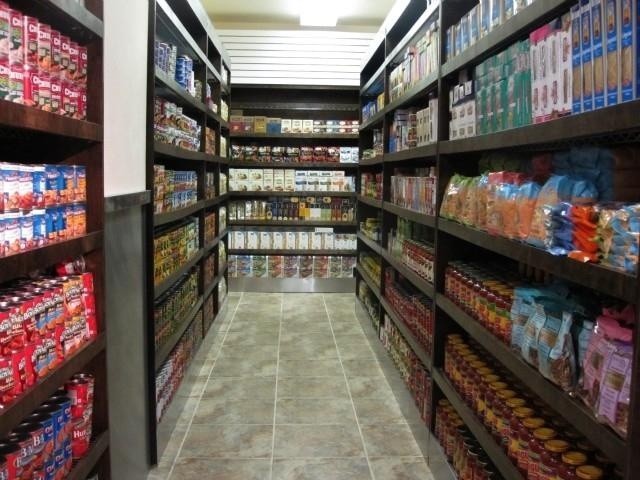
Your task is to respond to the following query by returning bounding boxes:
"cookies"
[510,285,633,438]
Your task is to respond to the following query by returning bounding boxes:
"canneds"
[444,260,527,344]
[443,335,600,478]
[385,315,433,429]
[387,286,432,356]
[388,240,434,283]
[434,399,496,478]
[0,255,97,480]
